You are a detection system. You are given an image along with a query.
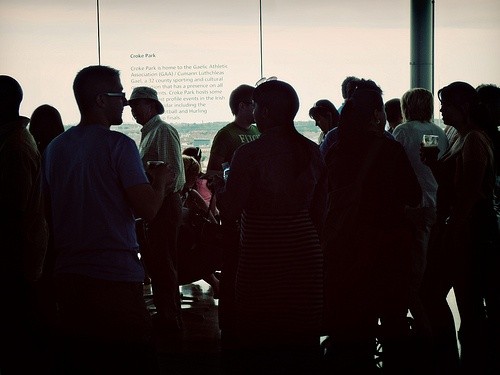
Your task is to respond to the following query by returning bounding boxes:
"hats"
[127,87,164,115]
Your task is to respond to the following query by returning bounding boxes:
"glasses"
[104,92,126,99]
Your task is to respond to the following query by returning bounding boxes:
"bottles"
[196,178,213,208]
[141,276,155,311]
[222,162,231,185]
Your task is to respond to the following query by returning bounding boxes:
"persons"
[205,85,262,211]
[127,87,183,329]
[0,76,47,375]
[208,80,329,375]
[168,147,218,284]
[29,105,64,154]
[319,77,424,375]
[385,82,500,375]
[309,99,339,144]
[39,66,154,375]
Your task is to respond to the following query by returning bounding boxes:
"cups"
[422,134,439,160]
[147,160,165,168]
[180,306,221,350]
